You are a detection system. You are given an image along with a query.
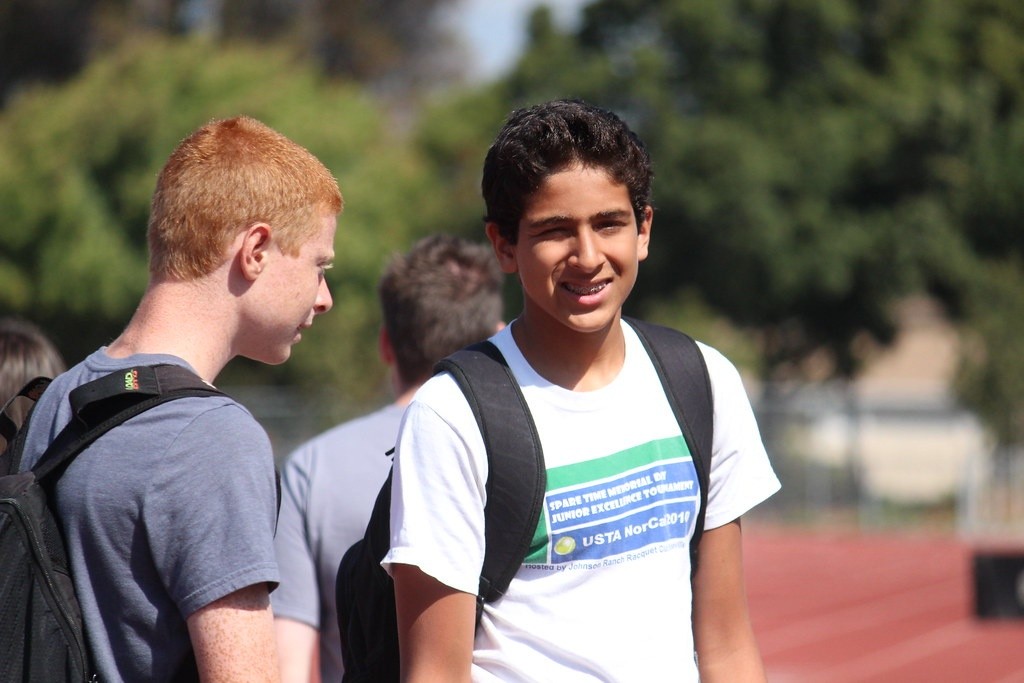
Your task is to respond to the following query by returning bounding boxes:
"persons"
[268,226,517,683]
[1,315,68,480]
[22,111,349,683]
[379,99,784,683]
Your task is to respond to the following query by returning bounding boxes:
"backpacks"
[1,362,233,682]
[333,316,715,682]
